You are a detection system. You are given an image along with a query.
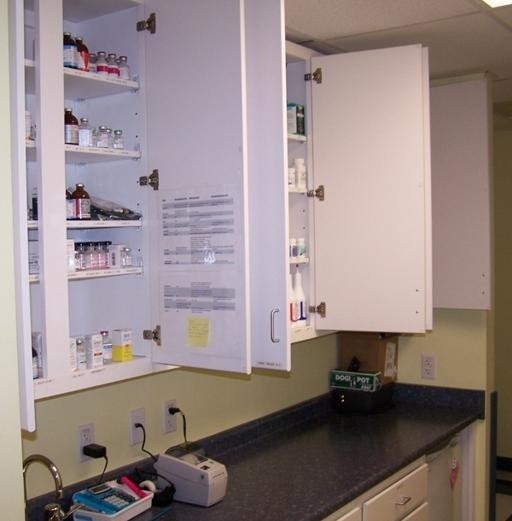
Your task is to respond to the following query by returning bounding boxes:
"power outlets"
[128,407,149,449]
[77,421,98,463]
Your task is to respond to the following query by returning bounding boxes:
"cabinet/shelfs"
[335,447,457,521]
[424,47,491,332]
[248,40,424,374]
[21,0,251,433]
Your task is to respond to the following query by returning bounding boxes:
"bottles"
[121,246,133,267]
[63,108,124,149]
[288,156,306,187]
[288,264,307,327]
[63,30,132,80]
[289,238,305,258]
[31,180,90,220]
[76,242,111,270]
[100,328,113,364]
[31,344,40,379]
[77,336,86,369]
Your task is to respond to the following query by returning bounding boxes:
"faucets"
[23,452,67,501]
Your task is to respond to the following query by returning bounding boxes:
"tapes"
[139,479,156,491]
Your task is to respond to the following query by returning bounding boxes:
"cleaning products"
[290,270,306,326]
